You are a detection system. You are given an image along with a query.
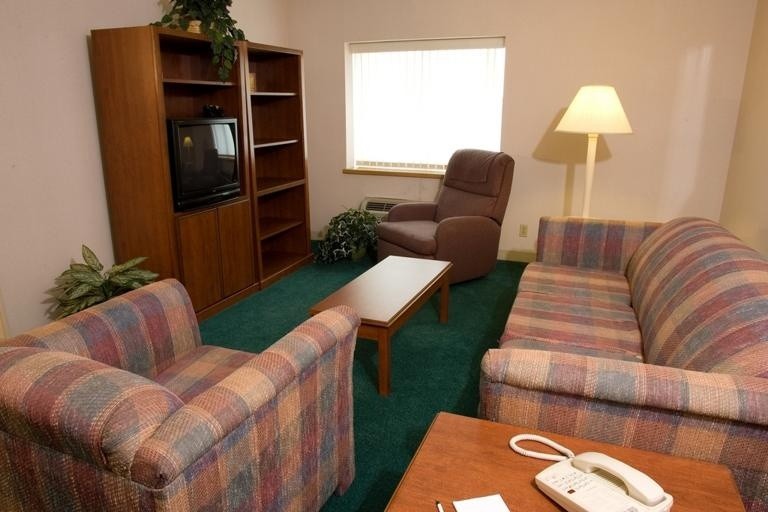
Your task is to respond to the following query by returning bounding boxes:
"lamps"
[554,85,634,219]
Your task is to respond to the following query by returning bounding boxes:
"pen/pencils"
[436,501,445,512]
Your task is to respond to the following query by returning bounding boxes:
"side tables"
[378,410,745,512]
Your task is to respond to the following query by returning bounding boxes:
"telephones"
[535,452,674,512]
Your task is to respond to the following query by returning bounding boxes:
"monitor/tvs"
[166,116,241,212]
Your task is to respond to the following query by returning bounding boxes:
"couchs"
[476,215,768,511]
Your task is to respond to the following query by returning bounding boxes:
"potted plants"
[149,0,245,84]
[311,206,379,269]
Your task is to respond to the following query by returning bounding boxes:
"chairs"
[377,149,516,285]
[1,278,363,511]
